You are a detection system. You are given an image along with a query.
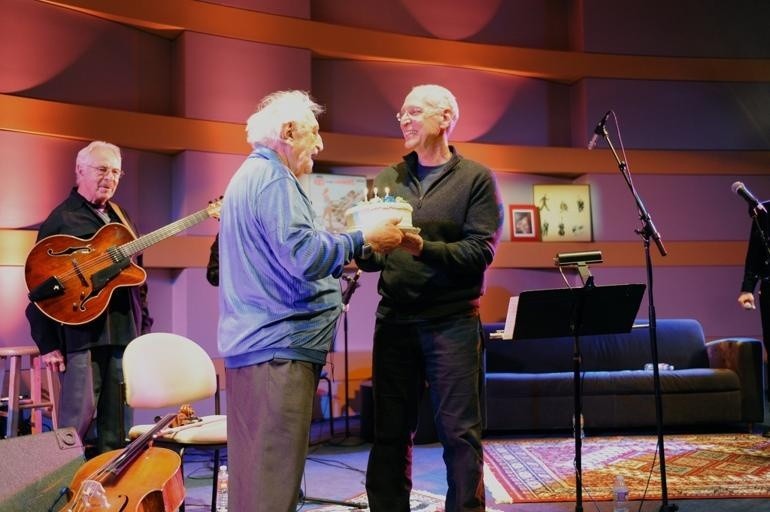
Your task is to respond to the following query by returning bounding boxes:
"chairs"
[118,333,227,512]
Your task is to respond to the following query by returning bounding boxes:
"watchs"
[361,230,371,261]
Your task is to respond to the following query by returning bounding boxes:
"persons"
[26,141,154,456]
[355,84,504,512]
[516,212,532,234]
[217,90,405,512]
[737,199,770,437]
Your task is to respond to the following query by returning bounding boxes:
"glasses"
[397,109,438,121]
[82,167,124,176]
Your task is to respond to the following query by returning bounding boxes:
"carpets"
[481,434,770,504]
[304,489,505,512]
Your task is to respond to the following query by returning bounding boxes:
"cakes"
[344,187,413,228]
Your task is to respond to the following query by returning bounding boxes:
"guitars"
[25,195,225,325]
[57,405,202,512]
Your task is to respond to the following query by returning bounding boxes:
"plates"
[343,226,424,240]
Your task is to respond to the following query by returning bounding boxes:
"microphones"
[744,302,753,310]
[731,181,768,215]
[587,111,612,151]
[341,270,362,312]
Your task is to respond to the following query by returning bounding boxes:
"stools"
[320,371,334,436]
[0,346,59,438]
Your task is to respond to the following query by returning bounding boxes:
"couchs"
[481,319,764,433]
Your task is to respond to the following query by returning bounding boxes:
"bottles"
[573,409,585,442]
[645,362,674,371]
[613,475,630,512]
[216,465,229,511]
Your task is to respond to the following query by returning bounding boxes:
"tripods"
[337,315,360,446]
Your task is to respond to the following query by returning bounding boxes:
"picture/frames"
[532,184,594,242]
[508,204,540,242]
